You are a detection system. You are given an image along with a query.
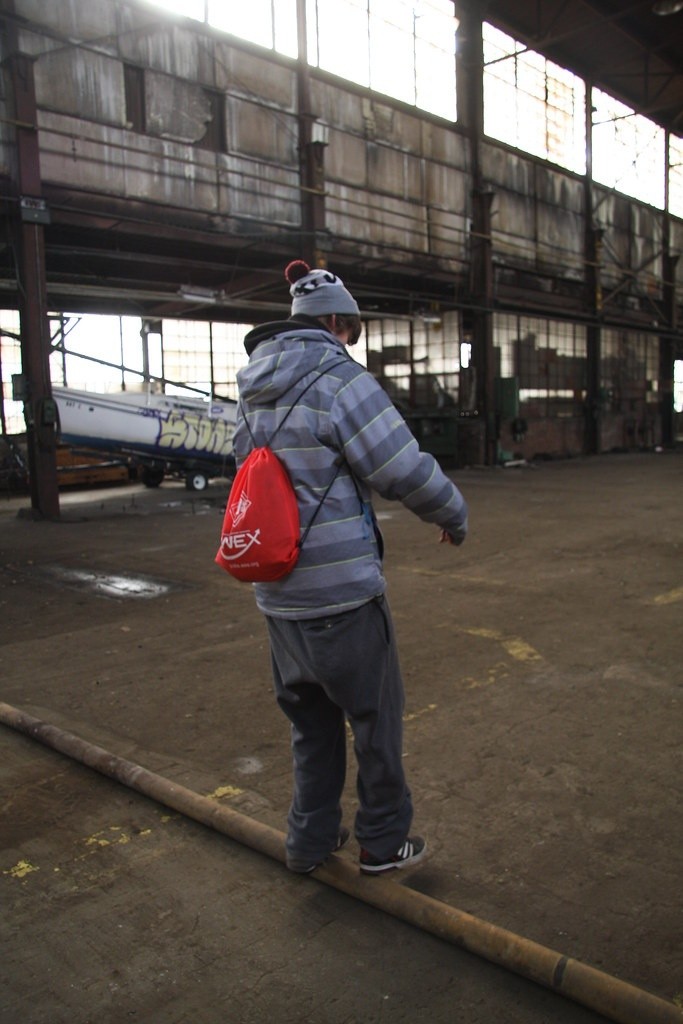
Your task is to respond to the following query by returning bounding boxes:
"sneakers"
[359,836,428,875]
[297,827,351,873]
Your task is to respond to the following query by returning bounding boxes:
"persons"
[228,257,482,877]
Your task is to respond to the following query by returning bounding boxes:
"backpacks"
[214,360,354,583]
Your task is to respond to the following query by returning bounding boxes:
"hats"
[285,260,361,317]
